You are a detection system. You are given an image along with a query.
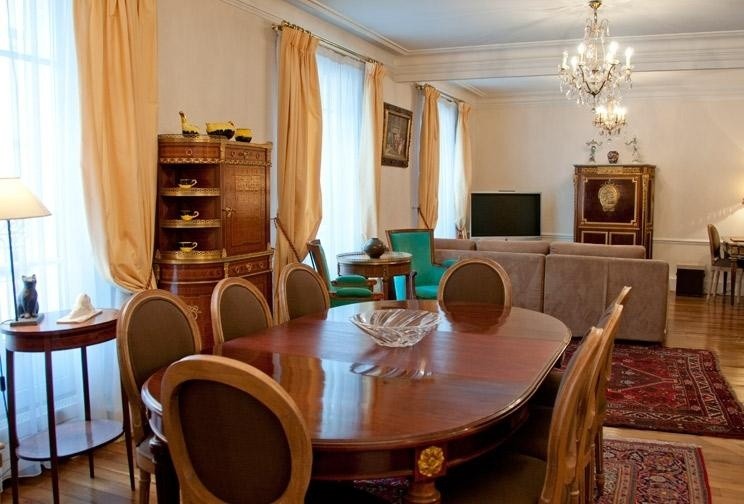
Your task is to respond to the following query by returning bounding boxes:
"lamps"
[0,172,60,328]
[589,97,629,138]
[553,0,637,113]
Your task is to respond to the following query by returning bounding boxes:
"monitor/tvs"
[469,189,542,241]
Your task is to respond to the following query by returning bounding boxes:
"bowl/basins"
[206,121,235,140]
[349,346,433,381]
[350,309,444,350]
[235,128,253,143]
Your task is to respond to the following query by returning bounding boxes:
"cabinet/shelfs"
[148,132,277,354]
[572,161,658,261]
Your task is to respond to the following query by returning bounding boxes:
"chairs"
[209,278,273,346]
[439,257,515,306]
[307,225,459,309]
[160,352,314,502]
[705,222,743,305]
[436,284,632,502]
[278,261,333,324]
[116,290,201,502]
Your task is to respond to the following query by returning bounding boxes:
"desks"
[139,299,571,502]
[717,234,743,307]
[0,305,140,504]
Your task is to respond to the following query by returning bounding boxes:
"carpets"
[584,431,715,504]
[550,331,744,443]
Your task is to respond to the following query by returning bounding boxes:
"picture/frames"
[379,99,413,170]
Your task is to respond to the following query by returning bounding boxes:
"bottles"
[364,238,386,259]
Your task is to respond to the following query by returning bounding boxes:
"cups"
[178,241,199,252]
[178,178,197,188]
[180,210,200,221]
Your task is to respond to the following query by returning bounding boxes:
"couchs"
[428,235,670,349]
